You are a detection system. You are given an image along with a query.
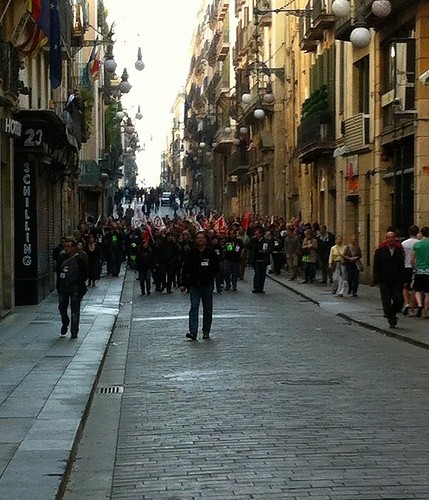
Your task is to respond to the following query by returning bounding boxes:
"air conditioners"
[342,114,371,150]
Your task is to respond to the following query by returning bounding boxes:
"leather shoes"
[389,324,395,328]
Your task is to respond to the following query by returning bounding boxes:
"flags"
[88,35,101,81]
[24,0,62,89]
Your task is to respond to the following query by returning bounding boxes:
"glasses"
[61,241,66,242]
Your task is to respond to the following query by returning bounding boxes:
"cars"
[161,192,173,206]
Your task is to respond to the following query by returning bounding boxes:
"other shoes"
[71,335,77,340]
[203,333,209,338]
[268,270,363,297]
[87,280,265,296]
[61,321,70,334]
[186,333,196,340]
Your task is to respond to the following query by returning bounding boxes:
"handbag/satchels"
[296,248,310,256]
[355,259,364,271]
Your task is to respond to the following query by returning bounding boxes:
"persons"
[63,89,82,117]
[410,226,429,319]
[379,226,405,248]
[372,232,406,329]
[55,240,87,338]
[53,186,363,298]
[401,225,421,316]
[181,232,222,340]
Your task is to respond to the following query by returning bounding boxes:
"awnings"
[14,110,79,171]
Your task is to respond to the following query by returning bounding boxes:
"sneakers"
[402,304,409,315]
[416,305,423,317]
